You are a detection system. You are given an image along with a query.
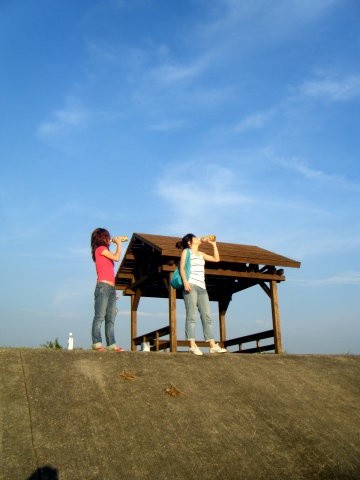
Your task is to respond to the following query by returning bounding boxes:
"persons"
[90,228,125,351]
[175,233,228,355]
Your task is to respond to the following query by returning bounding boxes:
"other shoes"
[92,344,107,351]
[210,343,227,353]
[107,344,124,351]
[188,345,203,355]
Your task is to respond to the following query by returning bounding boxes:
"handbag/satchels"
[171,248,191,289]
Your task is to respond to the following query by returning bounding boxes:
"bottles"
[67,333,74,350]
[109,236,128,242]
[199,235,216,243]
